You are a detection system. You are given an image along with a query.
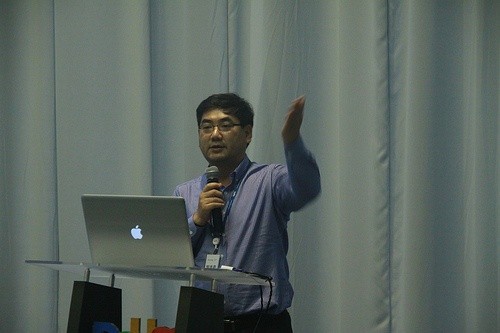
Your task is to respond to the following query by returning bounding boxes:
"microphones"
[205,166,224,236]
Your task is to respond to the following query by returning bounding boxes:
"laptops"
[80,195,202,270]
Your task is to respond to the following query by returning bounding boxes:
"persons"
[173,93,321,333]
